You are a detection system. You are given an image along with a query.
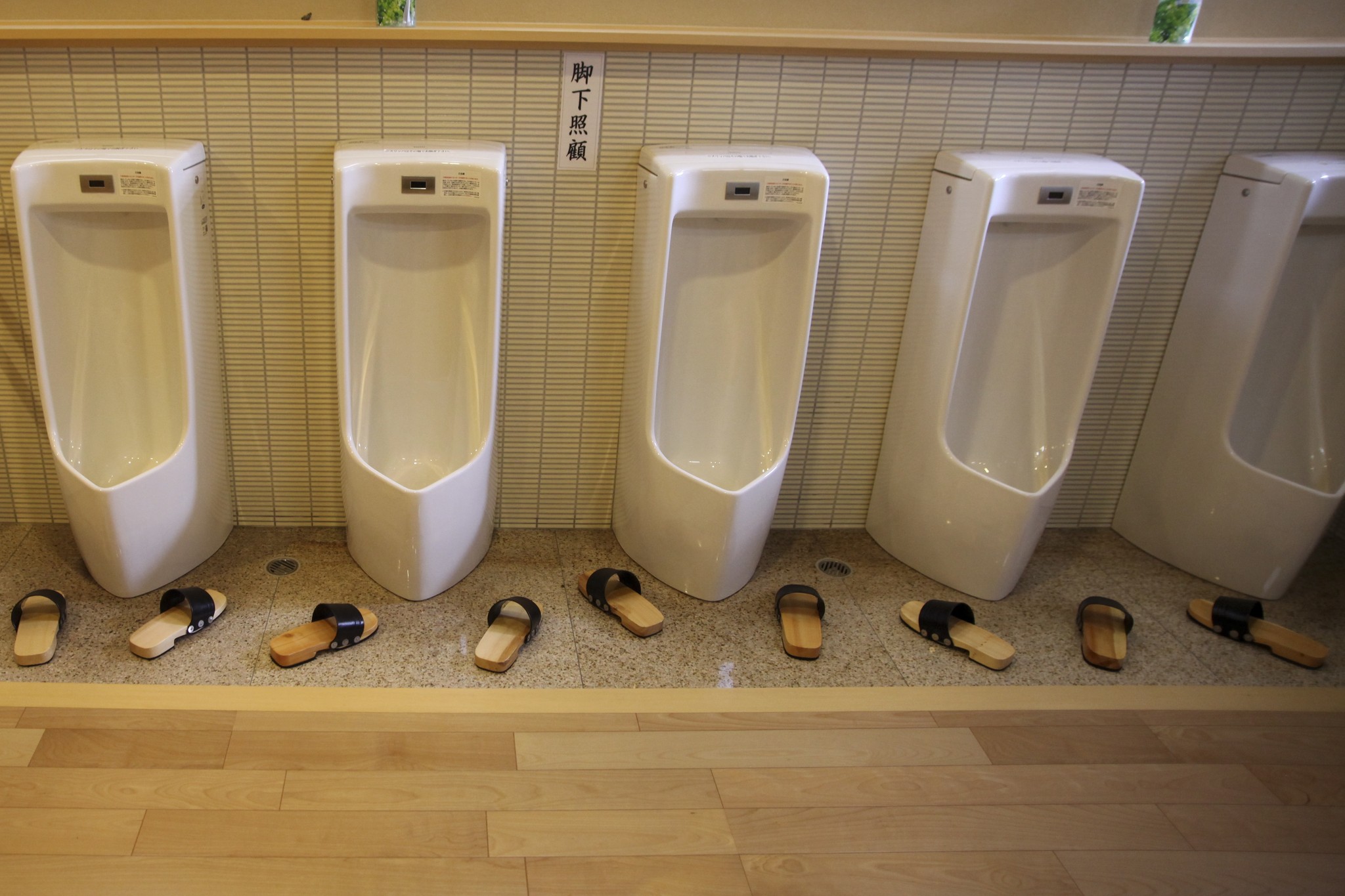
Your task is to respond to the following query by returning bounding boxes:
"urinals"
[329,137,506,602]
[866,149,1145,605]
[9,136,237,597]
[1111,150,1345,600]
[609,139,833,600]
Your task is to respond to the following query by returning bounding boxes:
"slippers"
[777,583,826,660]
[899,598,1015,670]
[127,587,229,660]
[270,602,379,671]
[578,568,666,638]
[1077,596,1135,672]
[1186,597,1329,670]
[474,595,545,675]
[10,588,67,668]
